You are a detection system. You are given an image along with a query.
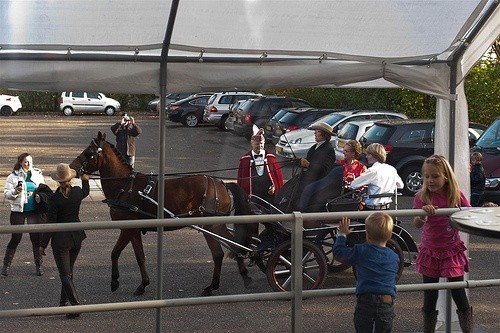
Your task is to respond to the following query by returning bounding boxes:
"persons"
[350,142,405,222]
[101,113,142,203]
[332,212,400,333]
[271,121,338,213]
[413,155,498,333]
[39,163,90,316]
[237,124,284,266]
[335,139,369,191]
[2,153,46,276]
[470,152,486,207]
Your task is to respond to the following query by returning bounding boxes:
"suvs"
[470,117,500,201]
[359,118,488,197]
[202,90,264,132]
[276,109,408,161]
[224,95,343,147]
[333,118,379,160]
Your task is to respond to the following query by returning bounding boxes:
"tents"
[0,0,499,333]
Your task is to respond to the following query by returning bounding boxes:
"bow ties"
[254,154,264,159]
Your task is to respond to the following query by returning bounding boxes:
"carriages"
[68,130,420,301]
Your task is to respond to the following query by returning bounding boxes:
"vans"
[59,91,121,116]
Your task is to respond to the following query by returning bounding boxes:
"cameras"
[124,118,130,123]
[18,181,23,187]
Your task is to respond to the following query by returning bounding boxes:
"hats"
[50,163,78,182]
[307,121,337,137]
[121,112,129,116]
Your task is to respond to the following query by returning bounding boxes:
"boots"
[59,274,83,318]
[457,306,475,333]
[1,247,16,276]
[421,307,439,333]
[33,248,45,276]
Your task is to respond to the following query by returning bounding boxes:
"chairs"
[409,131,421,140]
[299,163,346,209]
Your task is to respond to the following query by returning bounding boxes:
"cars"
[147,91,213,127]
[0,94,22,116]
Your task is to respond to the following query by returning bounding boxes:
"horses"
[69,131,255,298]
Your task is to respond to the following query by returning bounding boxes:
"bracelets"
[418,216,429,222]
